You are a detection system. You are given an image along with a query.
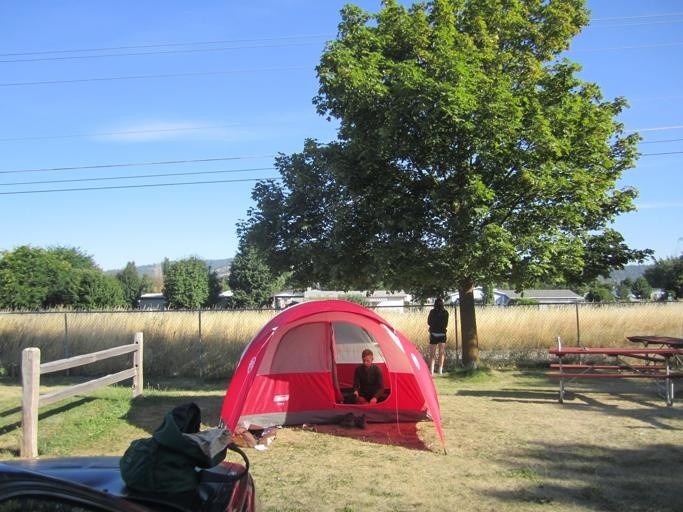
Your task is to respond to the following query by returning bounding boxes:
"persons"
[352,349,386,404]
[427,297,449,375]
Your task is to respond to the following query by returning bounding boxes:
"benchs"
[545,355,683,406]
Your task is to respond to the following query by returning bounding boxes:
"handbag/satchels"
[120,403,249,495]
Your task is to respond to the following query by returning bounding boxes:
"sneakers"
[344,413,354,426]
[431,368,434,373]
[438,370,442,373]
[358,415,367,429]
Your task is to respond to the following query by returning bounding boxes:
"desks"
[628,336,683,366]
[549,347,677,406]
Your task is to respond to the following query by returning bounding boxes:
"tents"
[218,299,446,457]
